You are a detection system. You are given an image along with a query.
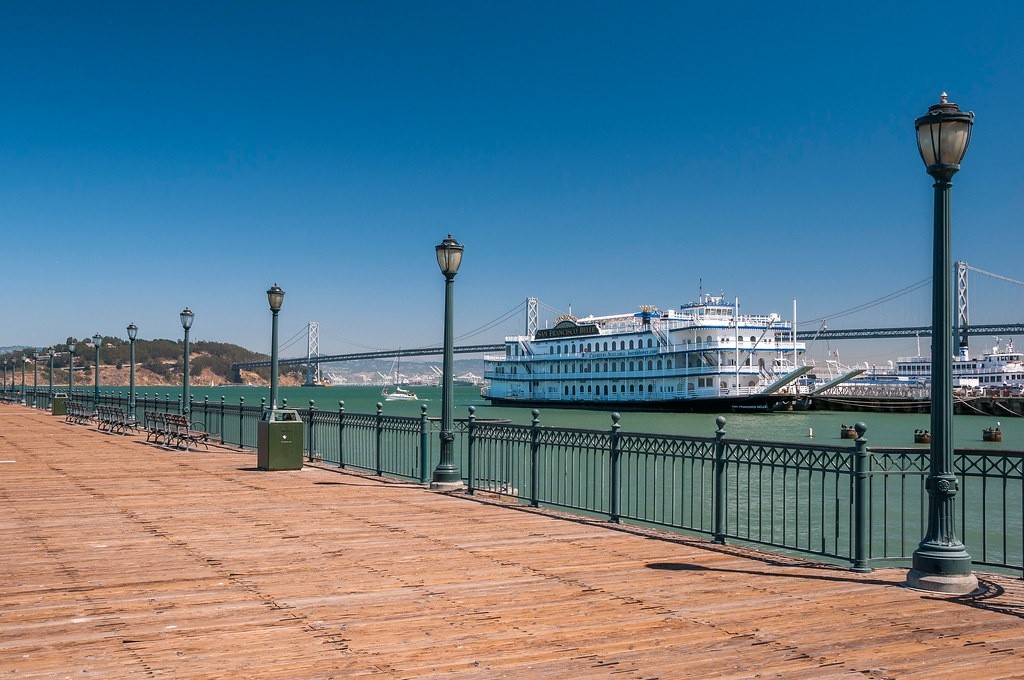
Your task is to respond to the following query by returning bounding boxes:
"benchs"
[0,393,17,405]
[144,411,184,444]
[96,406,122,433]
[73,402,97,425]
[65,401,85,424]
[164,413,210,451]
[108,407,141,435]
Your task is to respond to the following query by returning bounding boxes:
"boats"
[981,428,1003,442]
[799,335,1024,396]
[478,275,867,413]
[840,428,857,440]
[914,433,931,444]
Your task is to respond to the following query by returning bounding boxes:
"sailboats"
[380,346,419,401]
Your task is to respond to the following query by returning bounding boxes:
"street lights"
[903,89,982,597]
[66,340,77,416]
[10,356,18,403]
[91,330,104,421]
[179,305,196,429]
[31,350,40,408]
[126,321,138,428]
[265,282,286,421]
[2,357,9,404]
[47,345,56,412]
[20,353,27,406]
[428,232,466,494]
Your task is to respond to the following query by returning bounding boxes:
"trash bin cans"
[257,409,304,471]
[52,393,69,415]
